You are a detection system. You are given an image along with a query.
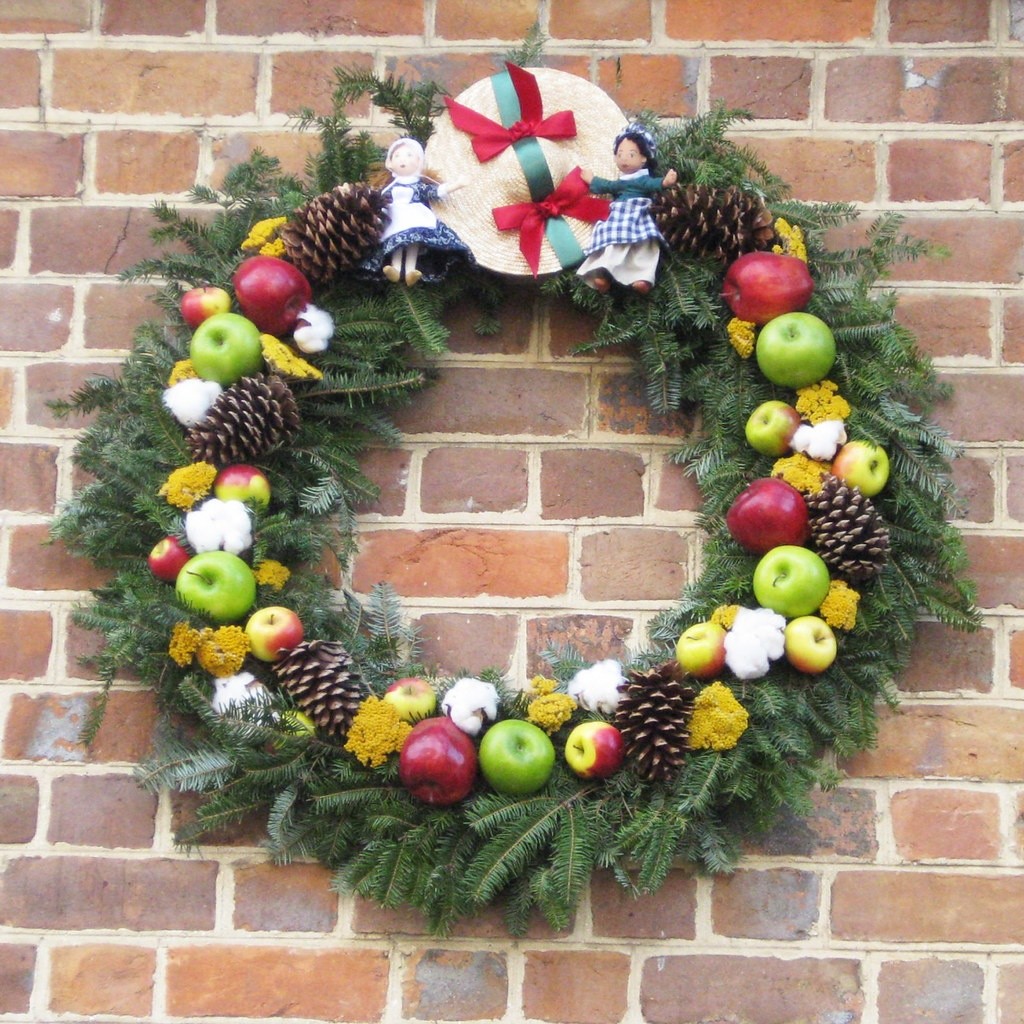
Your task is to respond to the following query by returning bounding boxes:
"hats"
[422,61,630,275]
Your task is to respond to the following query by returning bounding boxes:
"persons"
[576,123,676,295]
[375,138,475,284]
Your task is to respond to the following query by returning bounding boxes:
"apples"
[148,251,888,807]
